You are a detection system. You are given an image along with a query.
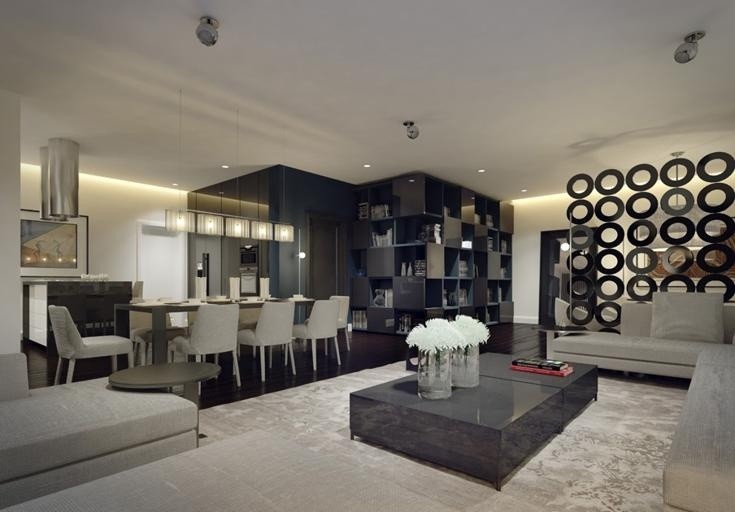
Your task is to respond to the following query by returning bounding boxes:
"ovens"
[240,270,258,294]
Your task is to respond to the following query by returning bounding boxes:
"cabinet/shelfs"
[423,172,515,329]
[345,169,422,335]
[23,281,141,350]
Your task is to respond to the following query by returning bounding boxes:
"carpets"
[1,353,689,510]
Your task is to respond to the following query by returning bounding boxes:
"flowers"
[405,318,465,354]
[451,314,492,349]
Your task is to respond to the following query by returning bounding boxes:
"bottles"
[374,289,385,307]
[407,262,413,276]
[401,261,407,277]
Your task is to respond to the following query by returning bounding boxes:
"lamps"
[165,87,293,243]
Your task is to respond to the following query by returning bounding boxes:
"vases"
[451,344,480,389]
[417,349,453,400]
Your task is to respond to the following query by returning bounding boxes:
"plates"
[202,299,230,302]
[415,273,423,276]
[288,298,305,301]
[163,301,182,304]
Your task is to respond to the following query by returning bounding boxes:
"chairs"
[330,295,353,353]
[231,302,297,382]
[553,298,621,334]
[176,303,242,399]
[48,305,135,386]
[292,300,342,372]
[130,310,259,373]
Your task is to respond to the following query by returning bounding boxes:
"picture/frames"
[21,208,90,279]
[636,205,735,289]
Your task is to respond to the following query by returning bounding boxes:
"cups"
[216,296,226,300]
[80,273,108,280]
[293,294,304,298]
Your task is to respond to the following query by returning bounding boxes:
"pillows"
[650,290,726,344]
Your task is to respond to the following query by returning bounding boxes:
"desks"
[111,297,320,374]
[109,360,224,447]
[531,325,587,360]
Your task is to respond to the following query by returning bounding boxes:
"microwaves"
[240,252,257,266]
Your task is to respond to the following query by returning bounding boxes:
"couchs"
[662,345,734,512]
[550,302,735,380]
[1,353,202,509]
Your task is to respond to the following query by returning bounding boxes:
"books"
[474,213,508,278]
[359,203,393,247]
[353,311,367,329]
[395,313,411,332]
[385,289,392,307]
[459,260,492,322]
[512,358,569,370]
[443,289,448,305]
[499,287,502,303]
[510,364,574,378]
